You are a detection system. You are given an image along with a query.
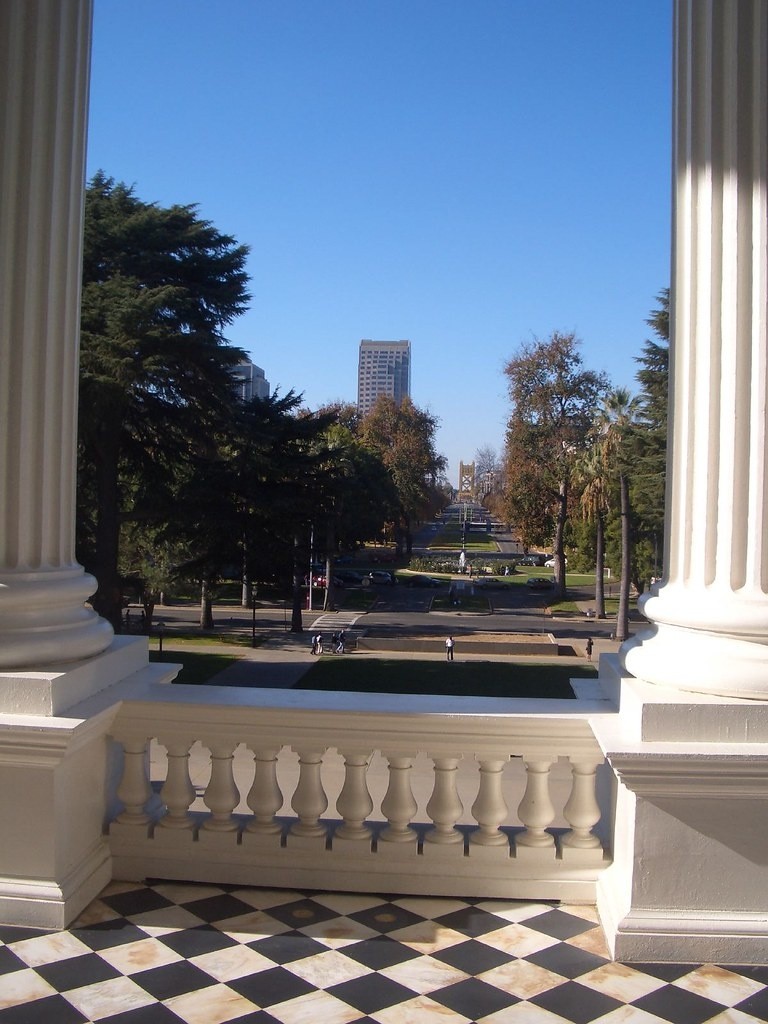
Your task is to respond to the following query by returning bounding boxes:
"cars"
[313,575,343,587]
[545,558,556,567]
[517,556,545,566]
[334,555,353,565]
[362,571,399,587]
[405,575,441,588]
[471,577,512,590]
[373,554,398,565]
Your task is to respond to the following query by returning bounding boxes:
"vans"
[527,578,553,590]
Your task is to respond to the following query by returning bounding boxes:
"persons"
[316,631,324,653]
[445,635,455,661]
[586,638,594,662]
[336,629,346,654]
[331,631,337,654]
[310,633,318,655]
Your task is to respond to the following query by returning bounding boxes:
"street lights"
[251,586,258,648]
